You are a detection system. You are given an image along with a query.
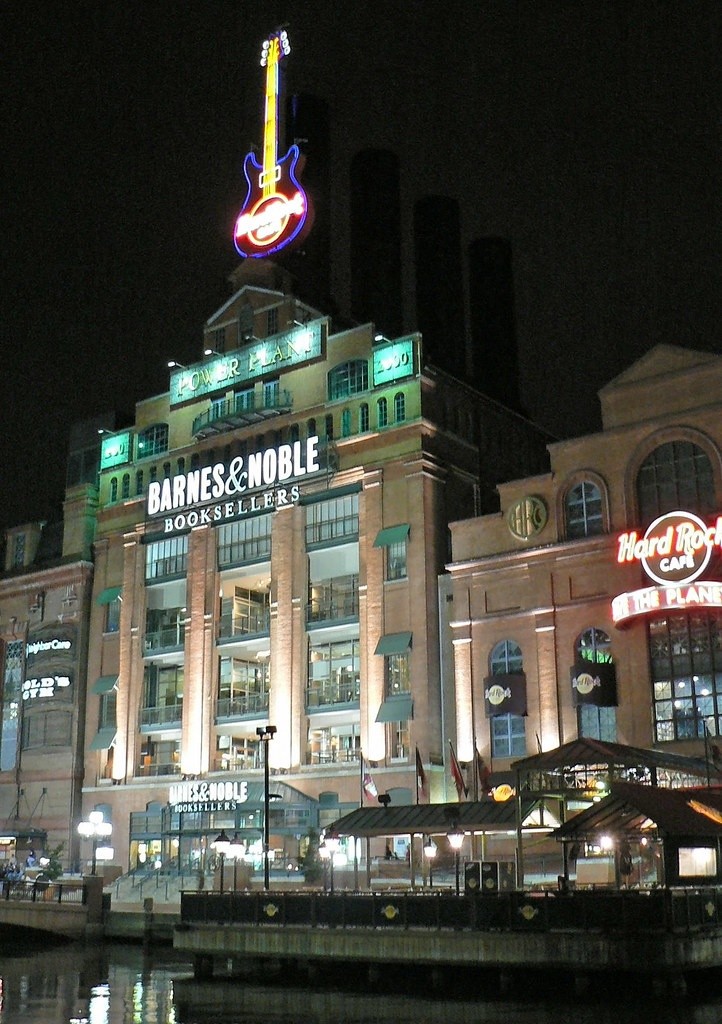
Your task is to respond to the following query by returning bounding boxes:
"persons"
[9,853,16,870]
[384,845,400,860]
[26,847,35,868]
[619,835,632,889]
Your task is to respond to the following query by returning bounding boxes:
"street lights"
[256,723,277,893]
